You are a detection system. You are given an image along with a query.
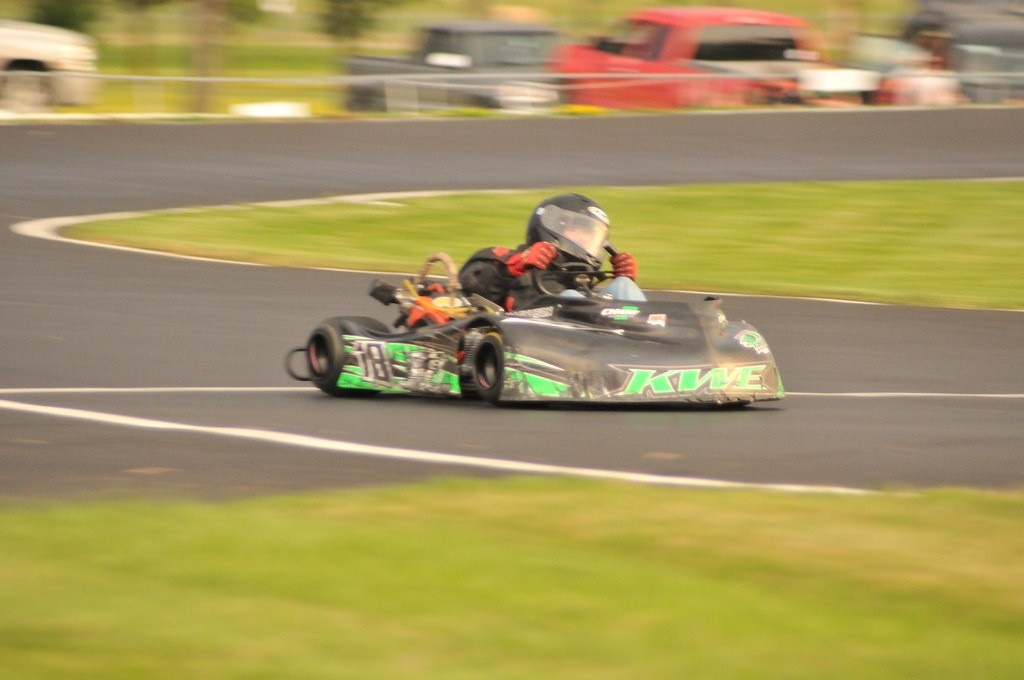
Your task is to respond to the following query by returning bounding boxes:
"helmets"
[526,193,612,272]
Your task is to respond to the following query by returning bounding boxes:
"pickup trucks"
[347,15,567,115]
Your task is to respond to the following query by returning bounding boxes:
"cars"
[0,22,104,110]
[844,1,1023,107]
[547,7,835,109]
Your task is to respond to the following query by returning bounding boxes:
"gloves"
[506,241,556,277]
[609,252,637,281]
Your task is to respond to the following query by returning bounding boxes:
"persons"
[861,17,973,105]
[458,192,647,314]
